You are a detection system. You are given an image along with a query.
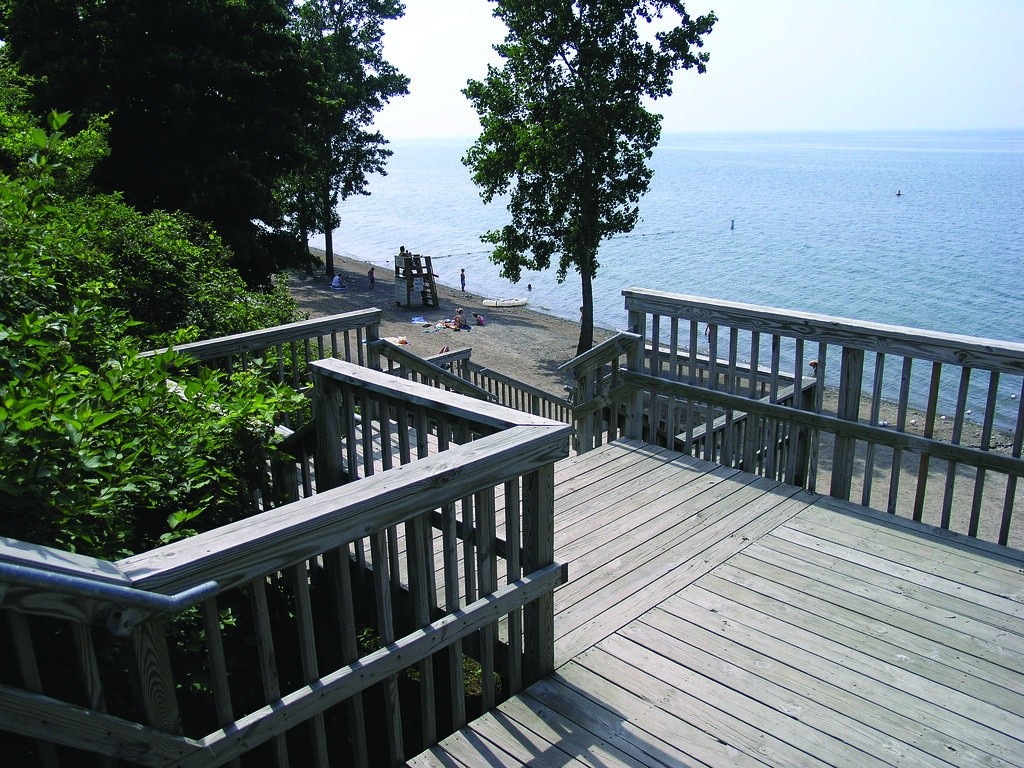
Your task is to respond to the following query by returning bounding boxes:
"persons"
[399,246,413,273]
[332,274,347,288]
[705,324,710,351]
[368,267,375,289]
[472,312,485,326]
[453,308,466,326]
[579,307,583,322]
[809,361,826,390]
[460,269,466,292]
